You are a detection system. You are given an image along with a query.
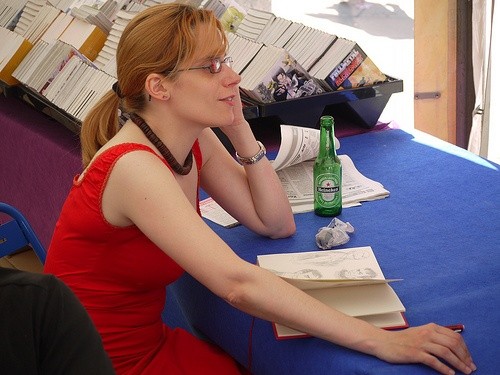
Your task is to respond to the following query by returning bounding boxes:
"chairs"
[0,202,47,266]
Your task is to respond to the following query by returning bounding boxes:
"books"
[0,0,392,124]
[255,245,408,340]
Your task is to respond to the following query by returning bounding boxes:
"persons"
[42,2,477,374]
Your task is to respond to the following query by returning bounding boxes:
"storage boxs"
[6,64,403,129]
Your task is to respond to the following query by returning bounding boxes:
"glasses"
[148,57,233,101]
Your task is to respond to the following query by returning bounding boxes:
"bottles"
[312,115,343,218]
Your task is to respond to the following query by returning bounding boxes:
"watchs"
[235,140,267,165]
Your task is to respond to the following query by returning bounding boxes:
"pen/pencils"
[445,325,464,332]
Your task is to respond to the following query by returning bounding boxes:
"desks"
[163,124,500,375]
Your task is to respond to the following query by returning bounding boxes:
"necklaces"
[128,112,193,176]
